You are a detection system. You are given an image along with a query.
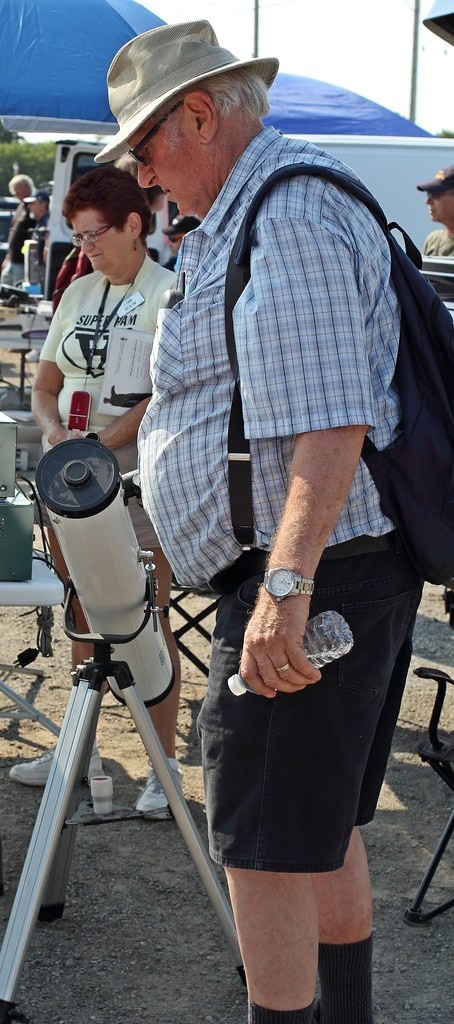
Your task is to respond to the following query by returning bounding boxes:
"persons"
[161,214,203,273]
[23,190,52,295]
[0,174,38,286]
[10,164,185,819]
[52,149,166,320]
[414,165,454,258]
[93,16,425,1024]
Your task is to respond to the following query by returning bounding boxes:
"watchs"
[263,566,313,603]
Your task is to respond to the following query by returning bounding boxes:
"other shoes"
[25,348,41,362]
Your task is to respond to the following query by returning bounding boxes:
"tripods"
[0,576,246,1024]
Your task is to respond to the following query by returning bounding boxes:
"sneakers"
[132,759,185,819]
[10,741,106,786]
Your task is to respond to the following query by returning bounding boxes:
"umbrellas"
[0,0,167,139]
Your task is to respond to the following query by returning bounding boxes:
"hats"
[22,190,50,202]
[162,216,201,236]
[416,164,454,193]
[94,19,279,164]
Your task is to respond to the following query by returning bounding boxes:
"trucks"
[38,135,454,321]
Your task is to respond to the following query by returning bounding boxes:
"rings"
[277,661,291,673]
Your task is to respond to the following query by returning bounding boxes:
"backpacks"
[224,162,454,585]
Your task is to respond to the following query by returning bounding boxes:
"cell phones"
[67,391,91,431]
[159,272,186,309]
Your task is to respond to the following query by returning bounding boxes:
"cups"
[91,775,113,815]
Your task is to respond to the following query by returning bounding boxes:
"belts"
[197,529,409,598]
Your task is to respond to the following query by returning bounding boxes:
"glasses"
[128,98,185,166]
[426,188,453,200]
[72,220,116,247]
[168,237,184,245]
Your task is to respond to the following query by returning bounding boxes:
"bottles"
[227,610,354,697]
